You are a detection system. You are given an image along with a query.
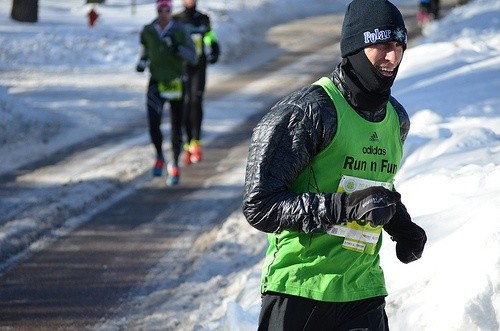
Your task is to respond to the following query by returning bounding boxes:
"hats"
[340,0,407,58]
[157,0,171,7]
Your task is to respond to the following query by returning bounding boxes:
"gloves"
[162,35,175,49]
[137,58,147,71]
[209,42,219,63]
[330,186,401,227]
[384,188,427,263]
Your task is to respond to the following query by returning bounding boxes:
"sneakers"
[177,144,191,167]
[153,158,164,176]
[189,138,201,163]
[166,162,179,185]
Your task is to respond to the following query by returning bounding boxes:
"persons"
[172,0,220,166]
[136,0,198,185]
[242,0,427,331]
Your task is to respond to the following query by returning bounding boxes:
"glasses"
[158,8,172,12]
